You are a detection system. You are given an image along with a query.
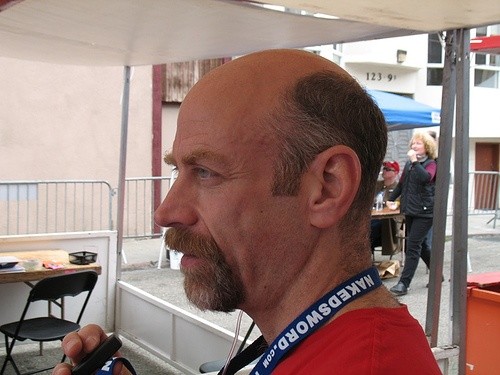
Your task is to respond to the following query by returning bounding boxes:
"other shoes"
[390,281,407,295]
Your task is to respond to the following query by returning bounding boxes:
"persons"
[386,133,438,294]
[52,49,441,374]
[371,161,401,252]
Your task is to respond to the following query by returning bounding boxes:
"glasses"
[383,167,394,171]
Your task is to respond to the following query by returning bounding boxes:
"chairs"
[0,270,99,375]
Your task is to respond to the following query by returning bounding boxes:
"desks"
[0,250,101,355]
[368,208,406,266]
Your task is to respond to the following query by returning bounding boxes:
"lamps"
[397,50,407,63]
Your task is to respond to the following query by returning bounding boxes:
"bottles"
[376,194,383,211]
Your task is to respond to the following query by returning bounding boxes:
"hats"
[383,160,399,173]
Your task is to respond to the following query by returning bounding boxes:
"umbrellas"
[364,89,441,132]
